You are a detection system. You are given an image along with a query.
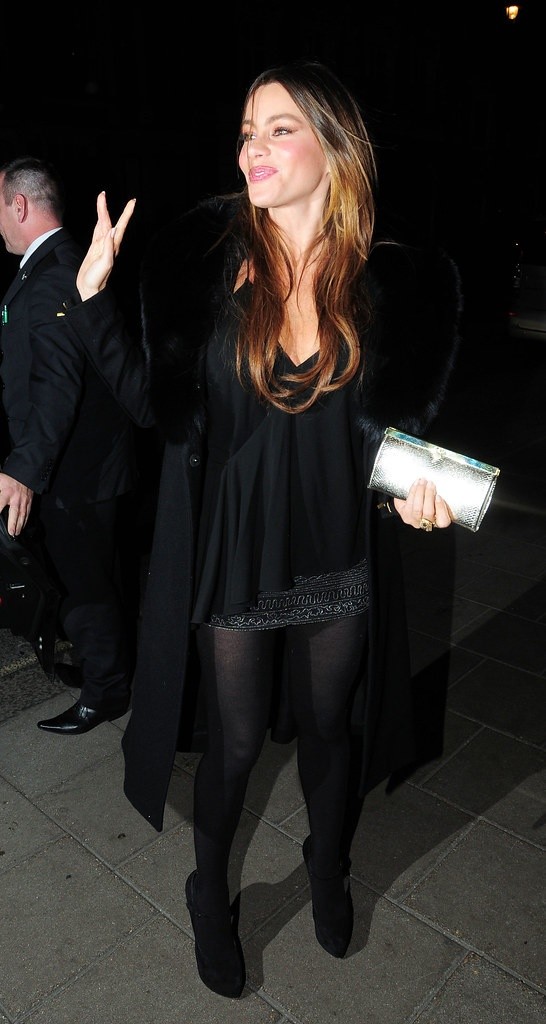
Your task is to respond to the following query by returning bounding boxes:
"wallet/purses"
[370,427,500,533]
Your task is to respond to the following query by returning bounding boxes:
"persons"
[75,57,468,993]
[0,160,142,735]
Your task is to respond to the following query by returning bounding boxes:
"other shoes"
[185,870,247,998]
[302,835,354,958]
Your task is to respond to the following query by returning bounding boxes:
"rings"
[418,516,435,531]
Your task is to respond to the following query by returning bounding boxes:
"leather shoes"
[54,661,84,688]
[37,698,129,734]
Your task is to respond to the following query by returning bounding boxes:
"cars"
[509,213,546,347]
